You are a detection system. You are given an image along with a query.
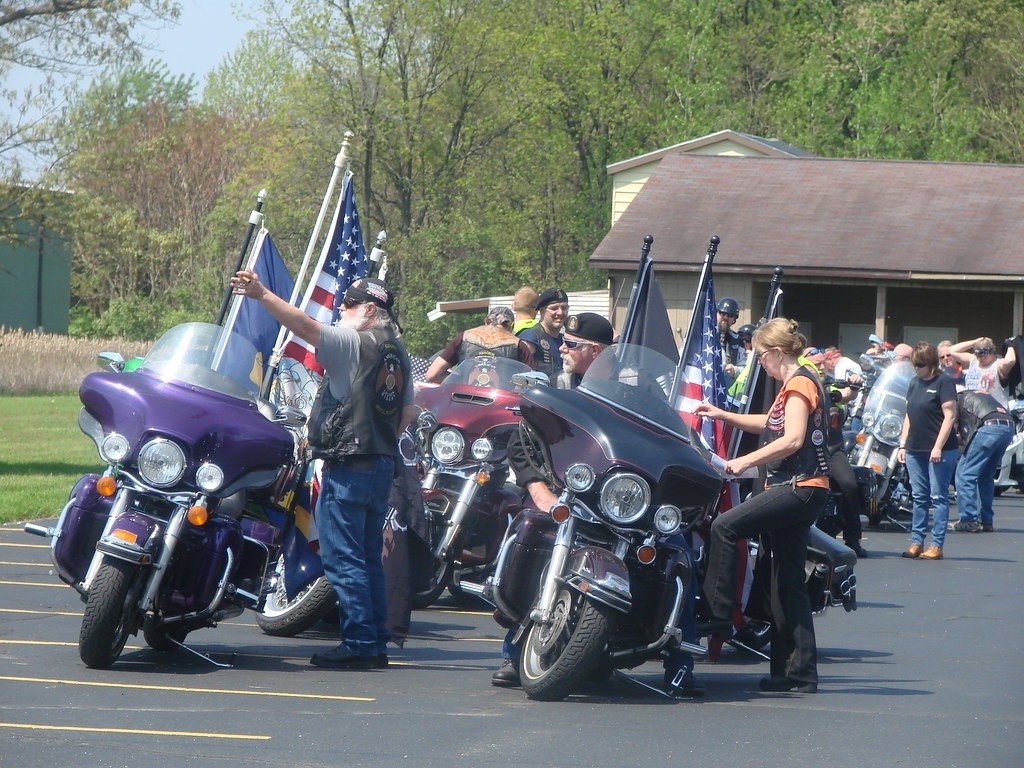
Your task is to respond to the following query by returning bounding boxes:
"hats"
[564,313,613,345]
[535,288,569,310]
[346,277,403,334]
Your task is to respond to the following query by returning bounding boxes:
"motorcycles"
[408,354,537,611]
[479,341,857,703]
[990,382,1024,497]
[22,320,306,671]
[253,552,334,637]
[834,333,917,533]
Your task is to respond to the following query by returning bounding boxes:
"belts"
[986,420,1013,426]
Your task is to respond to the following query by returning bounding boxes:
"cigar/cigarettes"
[241,275,252,284]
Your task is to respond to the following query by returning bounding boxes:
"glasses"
[755,347,787,360]
[913,363,927,368]
[738,335,751,343]
[343,297,361,309]
[804,348,825,358]
[719,311,734,318]
[561,336,592,350]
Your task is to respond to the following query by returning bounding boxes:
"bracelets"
[900,440,906,445]
[899,447,906,450]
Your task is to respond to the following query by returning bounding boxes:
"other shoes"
[310,641,389,669]
[844,542,867,558]
[947,520,993,533]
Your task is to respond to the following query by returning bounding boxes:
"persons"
[230,268,415,670]
[516,288,571,378]
[508,287,540,336]
[934,337,1016,533]
[897,342,960,560]
[713,297,756,408]
[803,338,897,424]
[491,312,707,695]
[426,307,536,390]
[689,316,831,693]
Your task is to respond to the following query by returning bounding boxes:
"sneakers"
[919,545,944,560]
[902,543,924,558]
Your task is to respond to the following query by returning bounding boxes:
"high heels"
[759,674,817,694]
[695,616,733,640]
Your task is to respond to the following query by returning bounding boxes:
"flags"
[730,287,785,505]
[674,268,749,641]
[616,255,681,401]
[280,168,371,380]
[210,227,303,394]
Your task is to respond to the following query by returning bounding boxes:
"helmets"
[716,298,739,316]
[737,324,756,337]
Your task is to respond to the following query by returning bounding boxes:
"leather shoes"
[663,669,708,696]
[492,658,520,687]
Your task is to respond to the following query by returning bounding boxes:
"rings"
[242,283,245,286]
